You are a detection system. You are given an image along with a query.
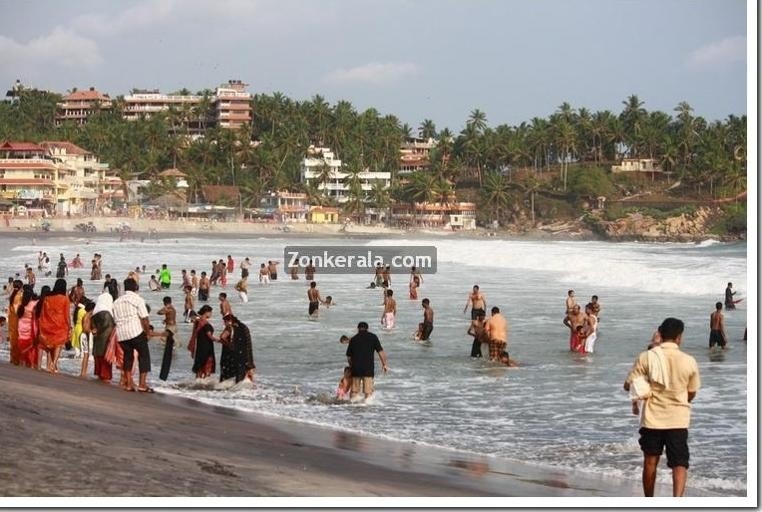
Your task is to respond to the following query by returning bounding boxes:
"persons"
[0,251,601,403]
[725,282,737,309]
[709,302,726,351]
[624,317,701,497]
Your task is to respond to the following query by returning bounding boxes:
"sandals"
[124,386,155,393]
